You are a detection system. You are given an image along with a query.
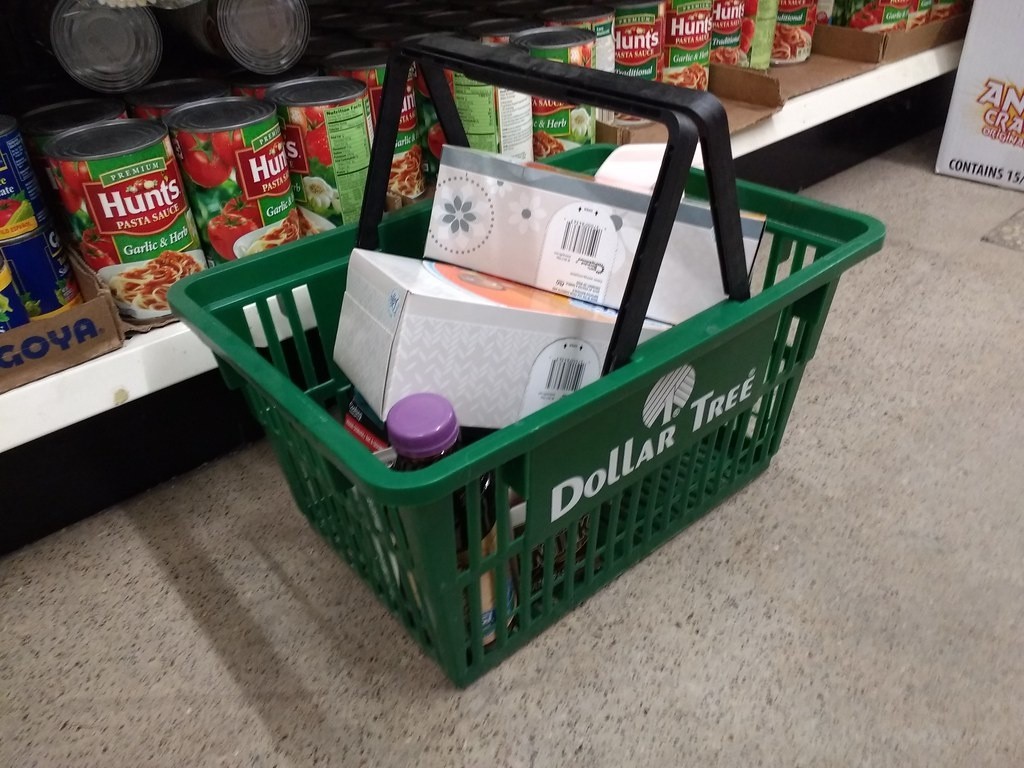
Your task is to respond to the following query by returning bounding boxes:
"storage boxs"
[933,0,1024,193]
[0,247,125,394]
[332,247,676,429]
[422,144,768,326]
[599,13,968,145]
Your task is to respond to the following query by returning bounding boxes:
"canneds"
[0,0,824,335]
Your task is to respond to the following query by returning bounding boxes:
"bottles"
[381,392,521,657]
